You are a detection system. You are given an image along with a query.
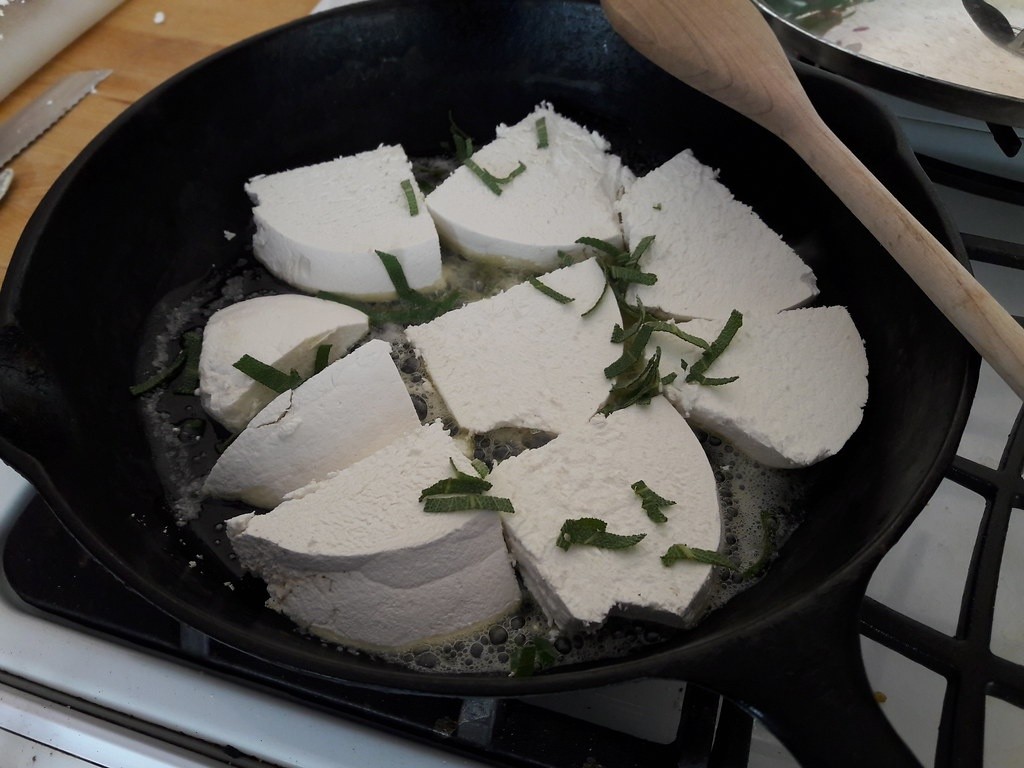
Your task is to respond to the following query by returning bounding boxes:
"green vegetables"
[128,117,787,674]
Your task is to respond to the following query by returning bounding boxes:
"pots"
[0,1,983,768]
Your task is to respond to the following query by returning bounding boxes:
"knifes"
[0,69,113,172]
